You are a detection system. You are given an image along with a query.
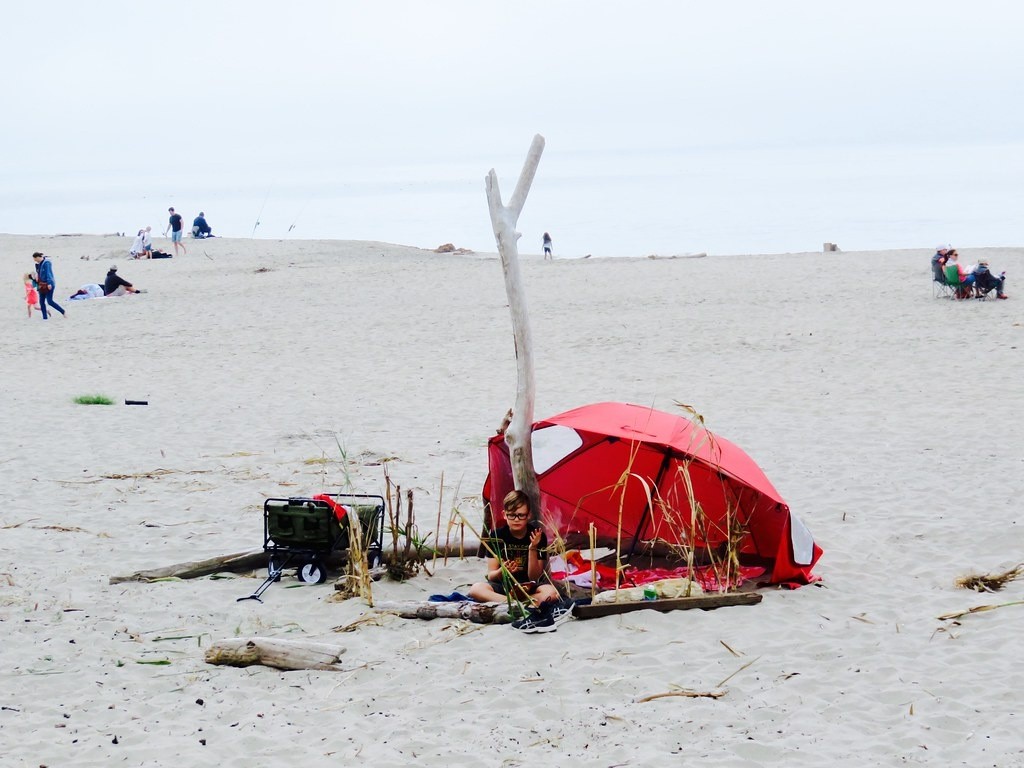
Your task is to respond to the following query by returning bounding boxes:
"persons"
[131,226,152,260]
[24,252,66,321]
[469,491,558,609]
[931,245,1008,300]
[70,265,140,300]
[192,212,215,237]
[164,207,186,256]
[542,233,553,260]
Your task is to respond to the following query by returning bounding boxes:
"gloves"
[1001,276,1005,281]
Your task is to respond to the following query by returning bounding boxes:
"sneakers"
[511,597,557,633]
[550,598,576,622]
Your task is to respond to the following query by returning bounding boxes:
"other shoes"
[975,295,985,299]
[998,294,1007,299]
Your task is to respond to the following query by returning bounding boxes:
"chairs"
[932,260,997,301]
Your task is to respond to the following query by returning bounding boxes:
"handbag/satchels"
[38,282,50,293]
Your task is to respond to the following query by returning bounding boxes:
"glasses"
[950,254,958,256]
[505,512,529,520]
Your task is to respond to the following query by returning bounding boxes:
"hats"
[936,244,950,251]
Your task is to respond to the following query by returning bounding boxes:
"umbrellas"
[476,402,823,590]
